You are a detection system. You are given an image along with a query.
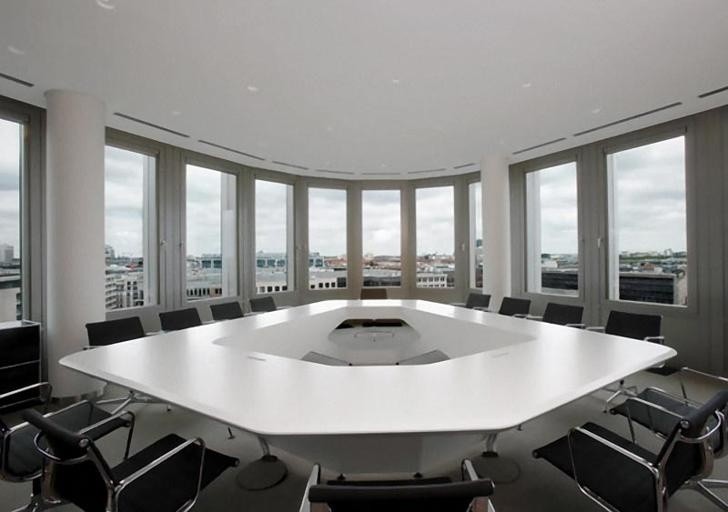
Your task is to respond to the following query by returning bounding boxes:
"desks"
[59,300,678,492]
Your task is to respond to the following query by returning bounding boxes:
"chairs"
[20,408,237,511]
[587,310,664,413]
[360,287,387,300]
[82,316,171,416]
[527,303,587,328]
[2,382,135,510]
[450,293,492,311]
[250,296,290,315]
[608,368,727,460]
[210,300,252,322]
[532,390,727,510]
[158,307,213,333]
[298,459,496,512]
[498,296,532,319]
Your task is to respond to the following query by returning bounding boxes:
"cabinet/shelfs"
[1,320,42,411]
